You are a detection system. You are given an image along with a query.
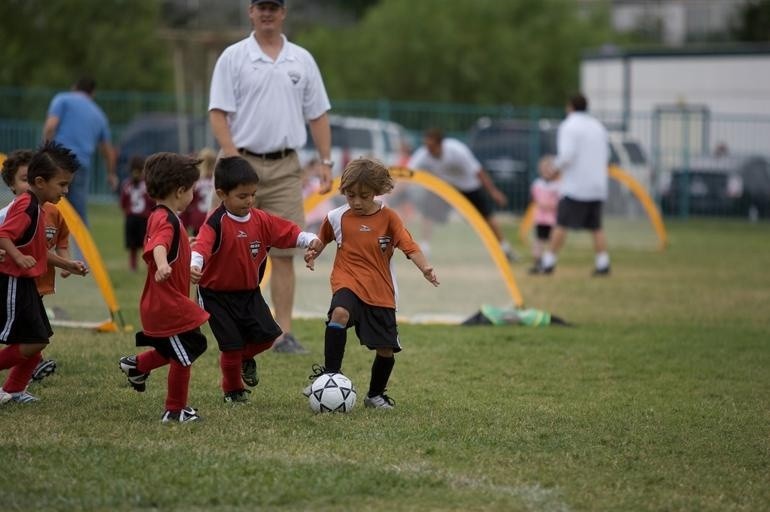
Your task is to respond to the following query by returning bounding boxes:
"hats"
[250,0,285,8]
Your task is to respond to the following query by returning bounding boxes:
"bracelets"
[321,159,334,168]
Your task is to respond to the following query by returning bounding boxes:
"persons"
[527,154,564,273]
[117,151,211,428]
[394,129,521,264]
[41,71,119,276]
[387,137,417,223]
[207,1,336,356]
[537,90,611,276]
[303,155,441,412]
[0,140,89,405]
[118,154,156,271]
[0,148,73,383]
[714,142,760,221]
[300,156,329,234]
[178,146,219,238]
[188,155,325,409]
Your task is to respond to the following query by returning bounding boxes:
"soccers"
[310,372,356,415]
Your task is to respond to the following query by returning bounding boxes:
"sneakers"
[0,387,43,413]
[29,360,57,381]
[119,355,150,392]
[272,333,311,354]
[302,364,344,397]
[223,389,258,415]
[240,357,259,387]
[364,389,395,411]
[159,407,205,432]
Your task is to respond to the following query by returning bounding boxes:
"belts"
[238,148,293,160]
